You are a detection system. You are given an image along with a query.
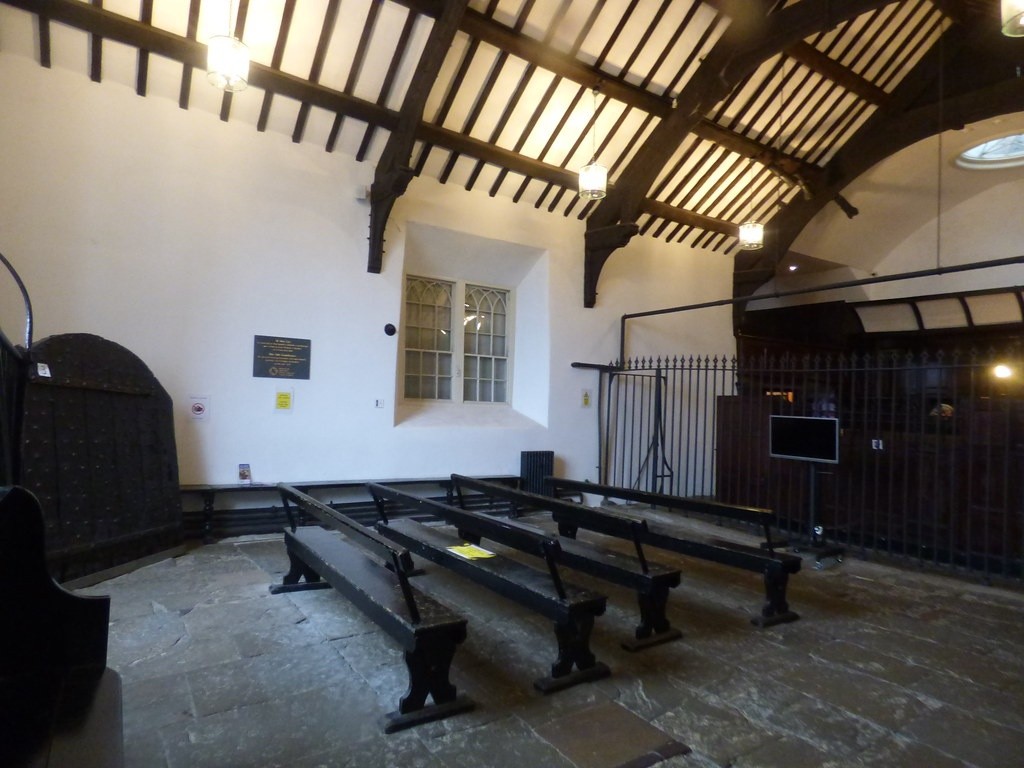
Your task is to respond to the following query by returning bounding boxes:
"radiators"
[520,450,554,496]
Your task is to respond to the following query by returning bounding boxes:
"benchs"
[179,475,523,543]
[269,473,803,735]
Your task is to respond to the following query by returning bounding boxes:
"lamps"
[738,158,764,251]
[1000,0,1024,37]
[578,87,607,200]
[206,0,250,93]
